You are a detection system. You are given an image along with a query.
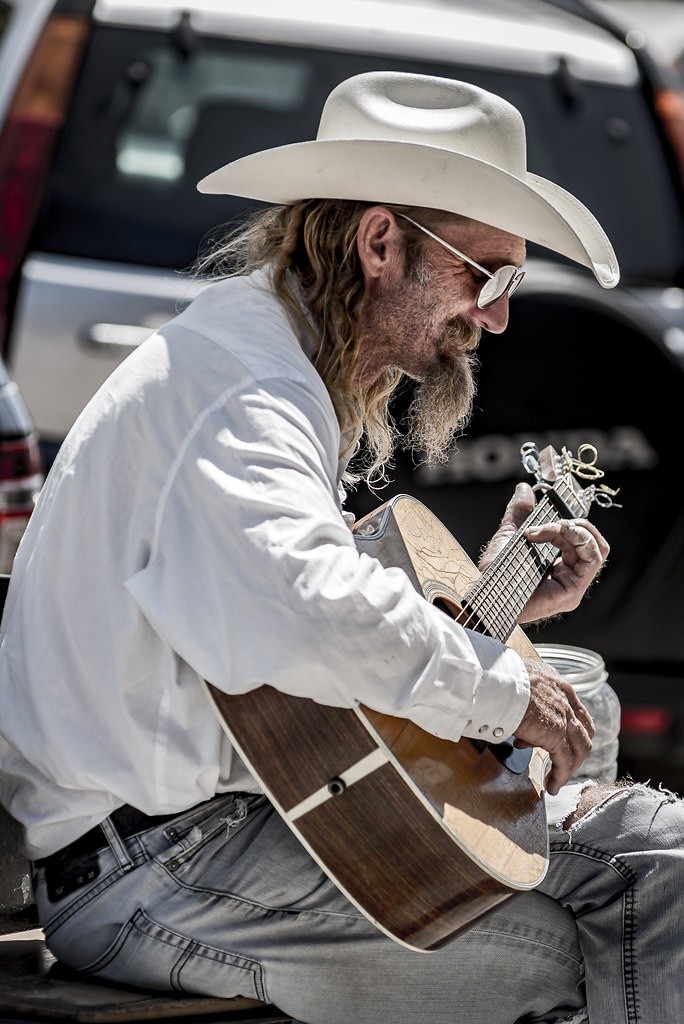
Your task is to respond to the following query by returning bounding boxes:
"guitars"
[197,440,626,965]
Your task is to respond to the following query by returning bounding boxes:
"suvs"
[0,0,682,499]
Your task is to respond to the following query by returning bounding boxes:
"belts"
[34,791,237,873]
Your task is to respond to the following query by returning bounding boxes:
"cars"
[326,264,684,797]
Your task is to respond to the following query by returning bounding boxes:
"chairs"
[182,97,294,197]
[0,577,277,1024]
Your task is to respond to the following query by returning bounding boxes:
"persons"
[0,70,684,1024]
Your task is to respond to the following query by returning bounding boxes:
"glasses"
[392,210,527,310]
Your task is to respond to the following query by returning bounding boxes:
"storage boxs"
[15,247,221,443]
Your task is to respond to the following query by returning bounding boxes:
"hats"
[197,70,620,290]
